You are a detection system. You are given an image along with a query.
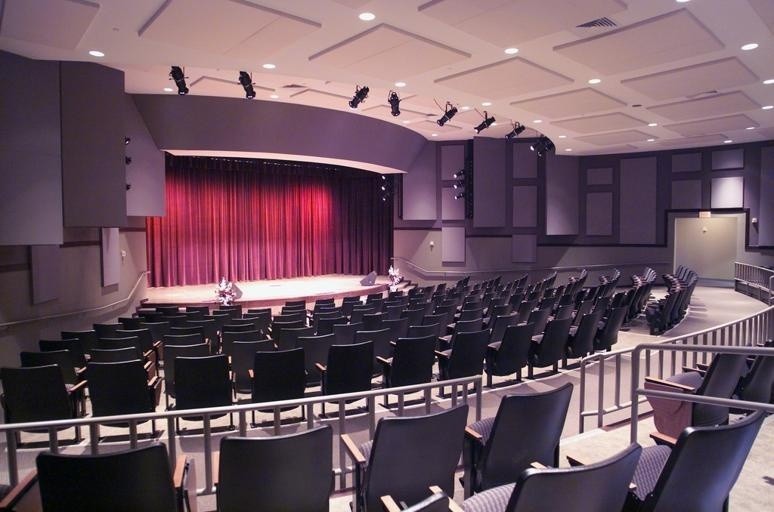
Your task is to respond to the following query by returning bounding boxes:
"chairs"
[698,338,774,411]
[370,299,386,312]
[89,349,154,384]
[186,319,217,344]
[567,410,761,512]
[165,352,235,437]
[118,316,142,332]
[429,438,641,512]
[422,284,435,301]
[456,302,484,321]
[439,318,484,349]
[422,313,453,336]
[134,309,163,323]
[451,264,699,292]
[453,292,467,298]
[556,303,575,319]
[282,308,308,325]
[231,338,274,397]
[205,314,232,332]
[248,308,272,317]
[139,321,169,341]
[114,329,161,368]
[232,317,264,330]
[162,315,188,328]
[458,382,574,502]
[387,305,405,321]
[0,365,87,449]
[33,439,196,512]
[162,339,210,409]
[596,306,628,355]
[409,298,428,312]
[305,312,343,329]
[395,296,411,307]
[592,296,611,313]
[466,295,485,304]
[214,310,238,320]
[431,294,450,303]
[413,302,437,315]
[273,314,303,323]
[172,325,205,344]
[248,347,308,429]
[353,326,391,378]
[283,305,306,311]
[435,283,448,297]
[39,337,90,361]
[365,293,383,305]
[484,323,534,389]
[20,349,86,383]
[413,292,427,297]
[388,290,403,301]
[567,314,596,363]
[343,301,364,315]
[489,312,522,340]
[575,300,592,325]
[188,305,210,314]
[310,306,343,313]
[518,300,536,323]
[307,304,337,314]
[340,296,359,311]
[376,334,435,410]
[407,287,419,296]
[434,327,491,399]
[332,322,366,344]
[401,307,426,326]
[317,316,351,336]
[528,318,571,379]
[377,489,446,512]
[91,322,122,338]
[381,301,402,314]
[482,292,500,304]
[632,293,692,334]
[221,330,260,356]
[391,323,443,348]
[58,330,101,354]
[485,297,506,318]
[538,297,557,317]
[432,304,458,318]
[482,304,515,326]
[96,338,160,373]
[155,306,176,317]
[339,402,469,512]
[266,328,316,354]
[352,308,375,324]
[267,320,308,335]
[84,357,164,443]
[645,349,746,435]
[508,294,526,309]
[381,318,409,338]
[348,303,374,322]
[243,311,271,327]
[223,324,257,334]
[526,307,551,336]
[307,298,332,312]
[213,423,335,512]
[446,308,485,334]
[220,304,241,319]
[296,333,335,384]
[184,311,202,321]
[314,340,372,419]
[363,311,388,331]
[285,300,305,309]
[164,333,202,359]
[438,297,463,307]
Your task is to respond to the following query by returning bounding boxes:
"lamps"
[505,124,524,140]
[474,115,495,134]
[381,196,389,203]
[381,174,388,179]
[388,90,401,118]
[127,157,132,162]
[168,64,188,95]
[237,70,256,100]
[529,136,545,151]
[124,136,131,145]
[539,143,553,157]
[126,183,130,188]
[383,185,391,189]
[347,84,368,110]
[454,180,466,190]
[437,106,456,128]
[456,194,465,200]
[452,169,464,178]
